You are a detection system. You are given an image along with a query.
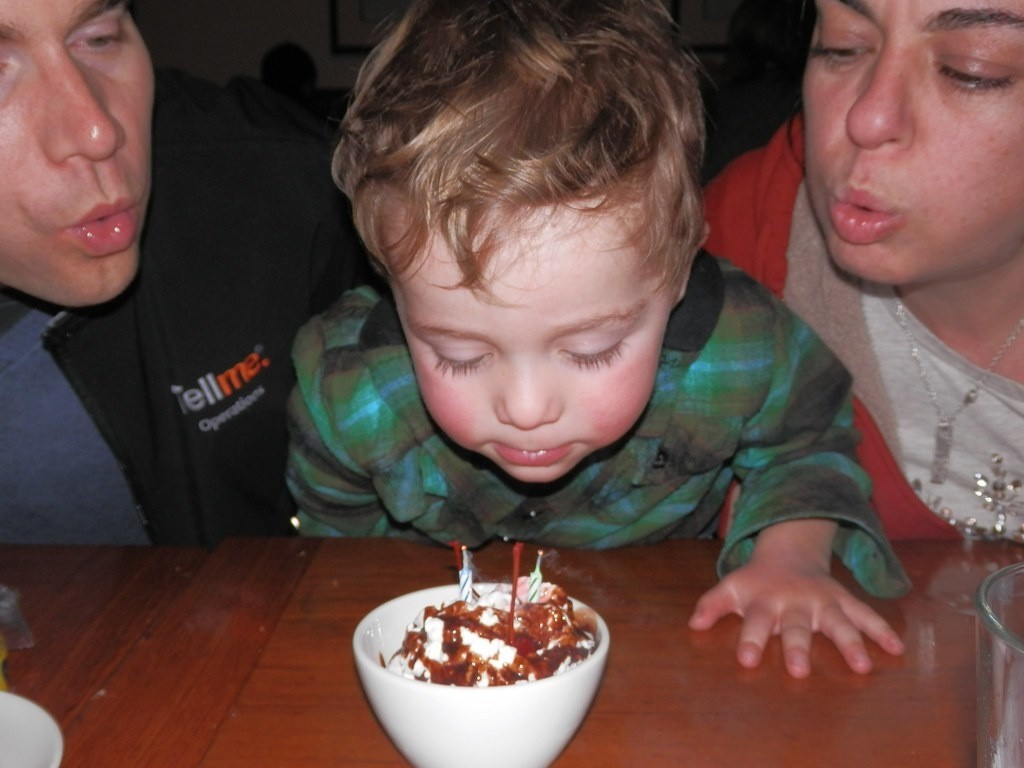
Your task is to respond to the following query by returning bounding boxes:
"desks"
[0,536,1024,768]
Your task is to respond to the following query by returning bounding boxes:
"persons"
[0,1,297,543]
[285,0,911,676]
[700,1,1024,542]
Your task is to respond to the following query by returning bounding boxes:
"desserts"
[385,576,596,686]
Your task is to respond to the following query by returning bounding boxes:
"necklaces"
[897,301,1024,483]
